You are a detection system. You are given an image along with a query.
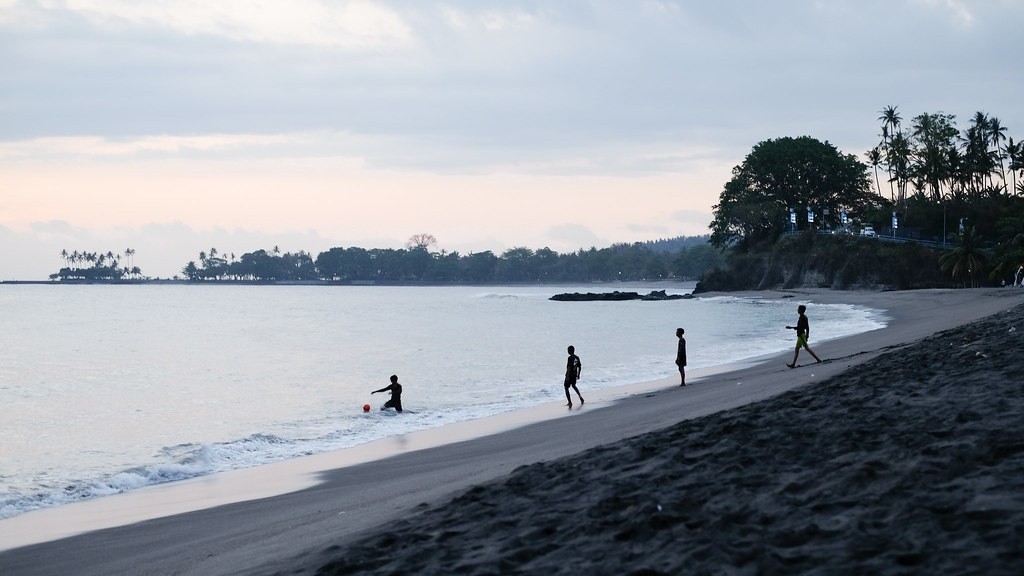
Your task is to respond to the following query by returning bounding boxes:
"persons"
[1013,264,1024,288]
[1001,278,1005,288]
[784,305,823,369]
[562,345,584,405]
[674,328,687,386]
[370,374,403,412]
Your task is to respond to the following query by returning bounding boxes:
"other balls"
[363,404,370,411]
